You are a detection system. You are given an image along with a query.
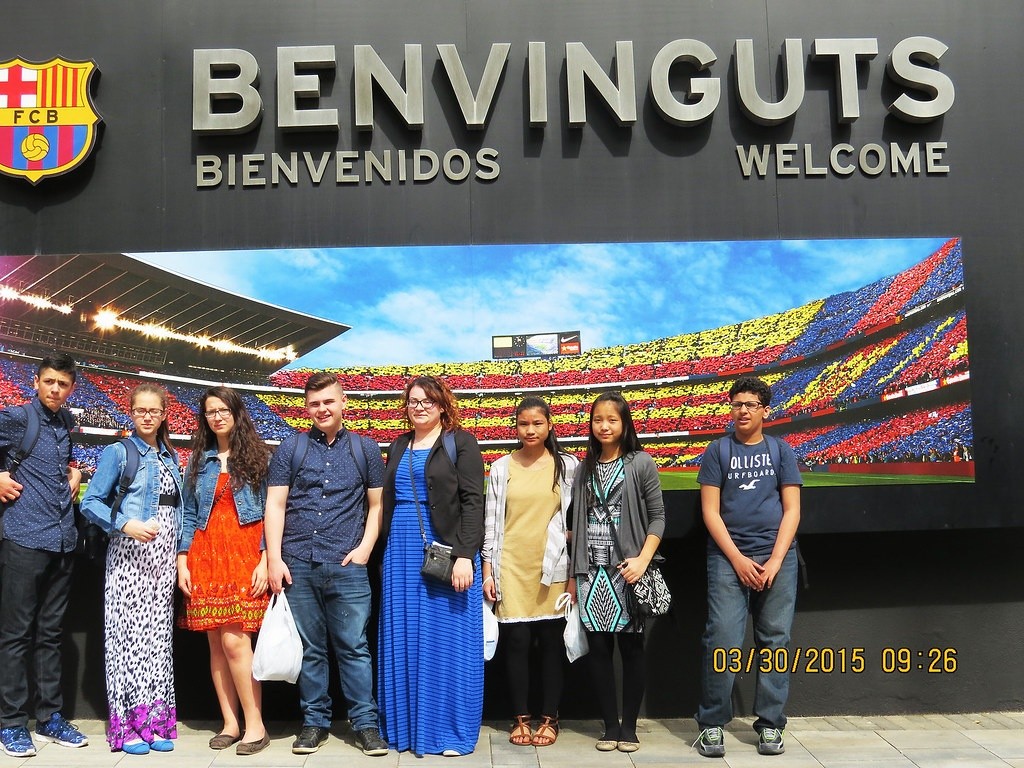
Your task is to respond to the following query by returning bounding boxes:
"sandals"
[509,714,531,746]
[532,714,559,746]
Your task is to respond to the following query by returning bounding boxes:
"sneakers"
[758,727,785,754]
[35,712,89,748]
[292,726,329,753]
[354,728,389,755]
[693,727,726,757]
[0,724,36,756]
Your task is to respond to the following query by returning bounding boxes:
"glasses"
[205,408,232,417]
[406,399,437,409]
[131,407,164,417]
[730,400,764,412]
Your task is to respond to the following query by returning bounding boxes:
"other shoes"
[596,731,617,751]
[122,742,150,754]
[209,728,242,749]
[618,731,640,752]
[236,729,270,754]
[149,739,174,751]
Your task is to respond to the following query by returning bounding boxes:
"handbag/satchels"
[420,541,454,585]
[252,587,303,684]
[482,576,499,661]
[623,562,672,619]
[555,592,590,663]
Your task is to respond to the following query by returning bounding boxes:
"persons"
[375,375,484,756]
[566,391,666,752]
[177,387,276,756]
[694,377,803,757]
[264,372,389,756]
[79,384,183,756]
[481,396,580,746]
[0,353,88,757]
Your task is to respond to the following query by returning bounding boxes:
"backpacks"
[83,437,139,572]
